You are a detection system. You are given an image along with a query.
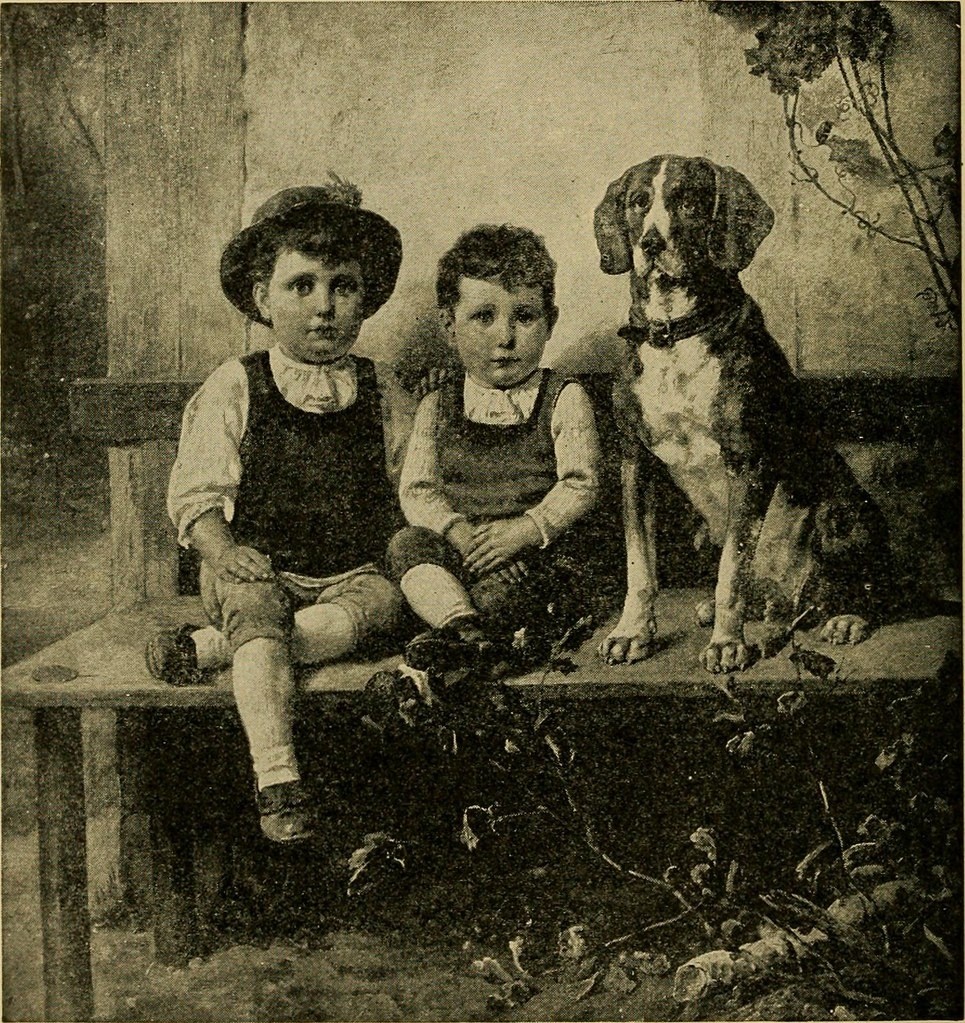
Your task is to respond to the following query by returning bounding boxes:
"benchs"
[1,364,963,1021]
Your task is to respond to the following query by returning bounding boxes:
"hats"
[218,185,403,329]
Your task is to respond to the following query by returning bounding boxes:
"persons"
[385,220,605,673]
[146,187,420,844]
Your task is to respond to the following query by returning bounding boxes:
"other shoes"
[144,623,229,684]
[403,618,513,691]
[359,671,422,771]
[253,775,318,844]
